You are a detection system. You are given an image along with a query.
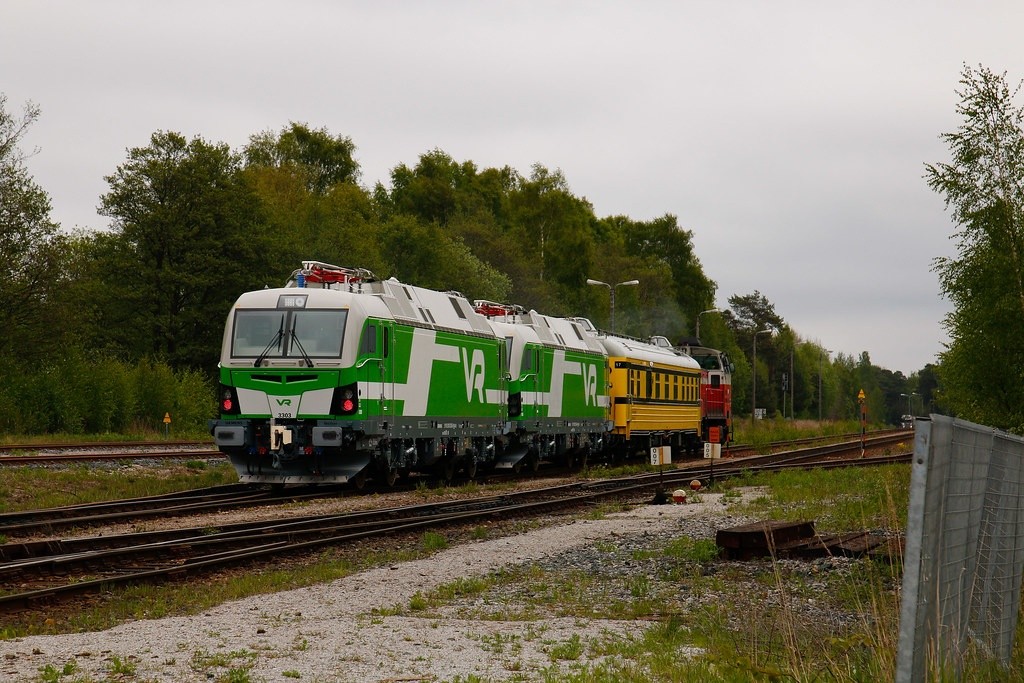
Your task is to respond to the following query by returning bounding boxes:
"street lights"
[900,393,917,416]
[790,342,808,421]
[752,330,772,421]
[818,350,834,420]
[587,279,640,333]
[695,309,720,340]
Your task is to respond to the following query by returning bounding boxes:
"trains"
[206,260,737,495]
